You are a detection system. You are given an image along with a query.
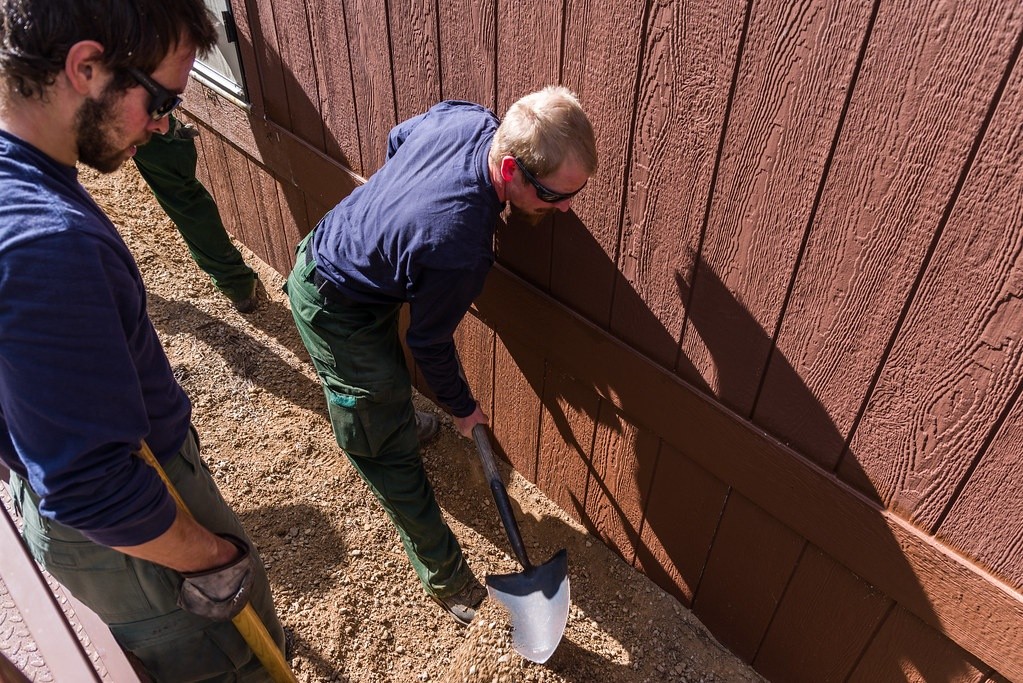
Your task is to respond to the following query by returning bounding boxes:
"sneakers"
[431,576,488,629]
[413,410,439,448]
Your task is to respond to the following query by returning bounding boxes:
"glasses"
[123,59,182,122]
[510,150,588,203]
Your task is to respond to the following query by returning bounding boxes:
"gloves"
[176,123,200,141]
[176,532,255,623]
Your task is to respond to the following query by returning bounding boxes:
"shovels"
[455,347,571,665]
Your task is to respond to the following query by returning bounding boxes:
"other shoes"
[233,264,258,314]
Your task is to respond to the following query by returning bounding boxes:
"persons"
[0,0,287,683]
[133,120,258,312]
[278,88,600,624]
